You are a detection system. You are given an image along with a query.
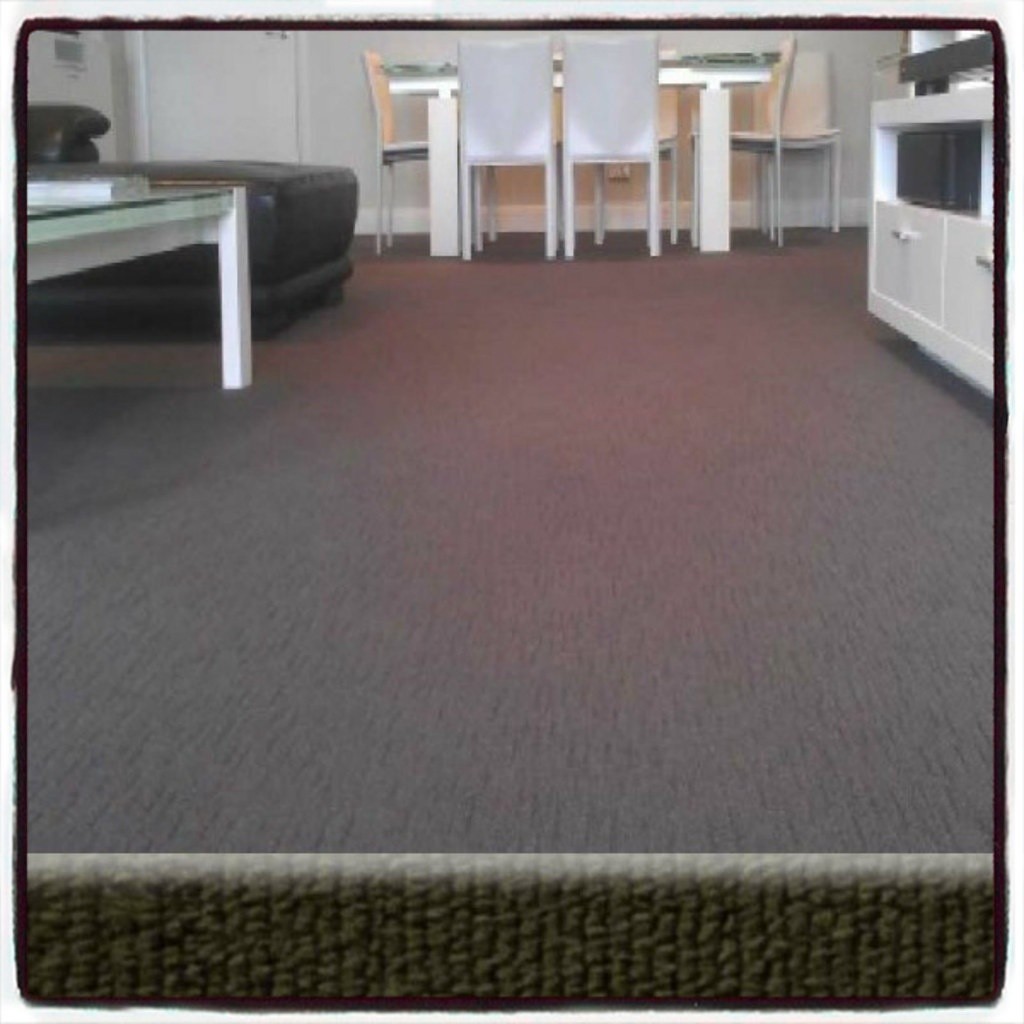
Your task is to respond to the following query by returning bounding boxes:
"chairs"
[690,33,798,250]
[592,49,684,246]
[561,30,663,258]
[363,50,497,256]
[457,33,561,261]
[760,48,843,243]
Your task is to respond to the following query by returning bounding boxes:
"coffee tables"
[28,180,253,391]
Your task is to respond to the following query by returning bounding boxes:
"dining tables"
[386,65,772,253]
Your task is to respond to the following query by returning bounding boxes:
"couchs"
[28,105,358,343]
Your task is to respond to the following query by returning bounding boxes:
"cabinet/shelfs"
[865,89,994,400]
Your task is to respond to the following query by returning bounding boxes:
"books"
[26,174,156,210]
